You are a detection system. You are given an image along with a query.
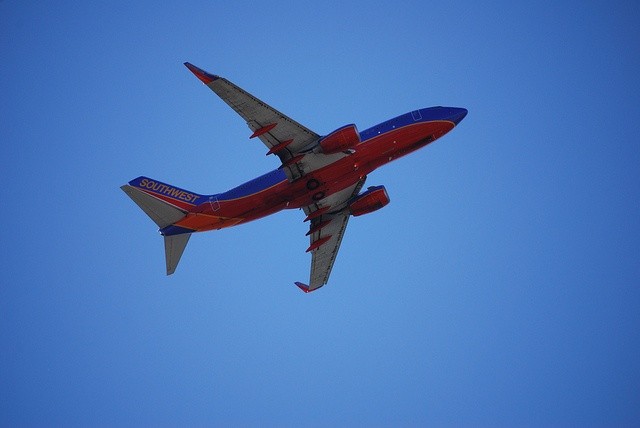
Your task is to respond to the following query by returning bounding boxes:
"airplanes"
[119,61,469,294]
